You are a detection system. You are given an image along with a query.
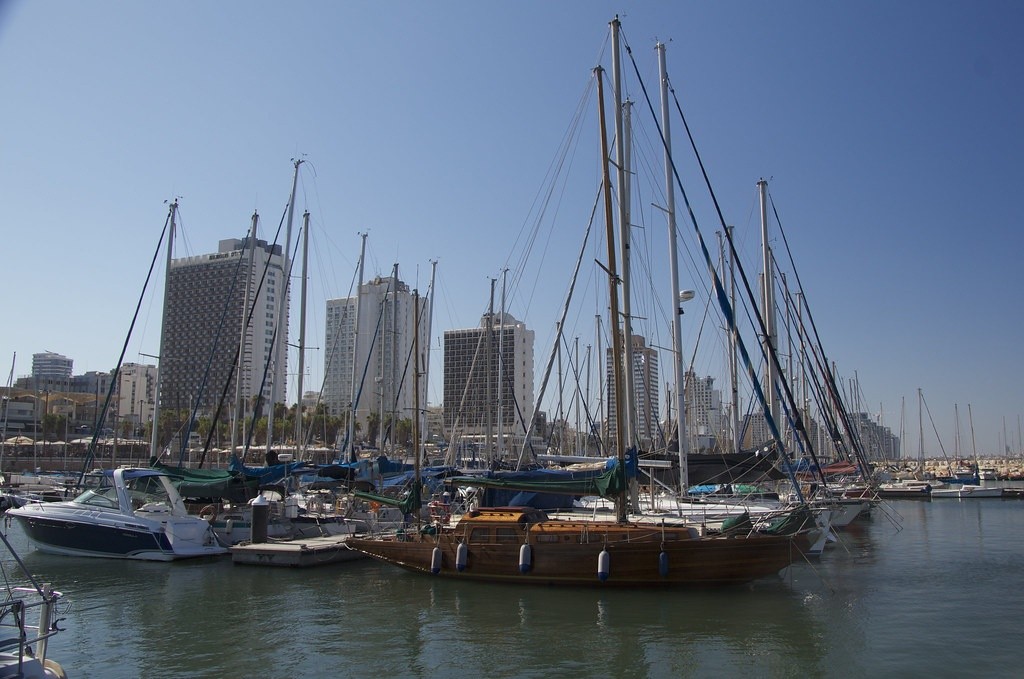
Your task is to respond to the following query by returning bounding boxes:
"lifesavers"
[307,496,324,514]
[428,501,451,524]
[200,504,218,523]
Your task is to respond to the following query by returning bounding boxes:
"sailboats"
[0,12,1024,679]
